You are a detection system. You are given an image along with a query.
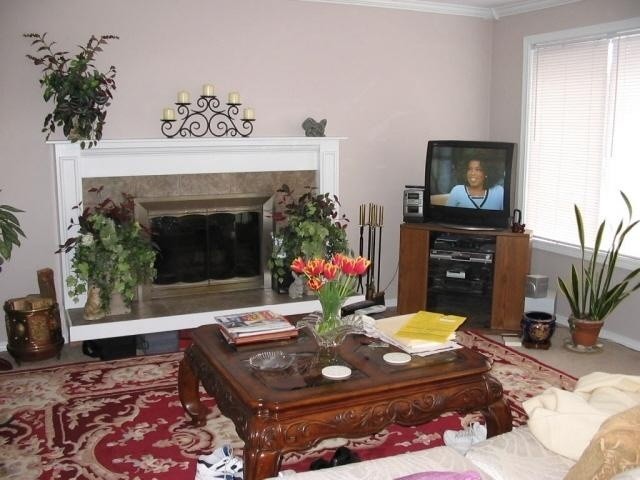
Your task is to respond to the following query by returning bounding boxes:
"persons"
[446,155,504,210]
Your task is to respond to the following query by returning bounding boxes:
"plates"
[249,350,295,369]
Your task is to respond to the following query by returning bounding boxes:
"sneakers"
[443,422,487,446]
[195,445,244,479]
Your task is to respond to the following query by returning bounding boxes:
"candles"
[242,108,253,118]
[227,92,240,103]
[162,107,173,118]
[201,84,214,96]
[177,90,189,102]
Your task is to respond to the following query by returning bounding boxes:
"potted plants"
[555,190,639,347]
[19,27,121,152]
[63,185,160,319]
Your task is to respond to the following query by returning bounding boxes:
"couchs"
[265,364,640,479]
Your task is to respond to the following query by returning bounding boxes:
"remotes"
[342,301,374,316]
[354,304,386,315]
[405,185,424,188]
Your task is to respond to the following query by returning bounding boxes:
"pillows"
[562,401,640,479]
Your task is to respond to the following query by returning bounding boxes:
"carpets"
[0,319,583,480]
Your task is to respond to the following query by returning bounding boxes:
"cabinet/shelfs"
[396,220,534,338]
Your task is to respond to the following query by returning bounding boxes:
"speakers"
[403,188,424,224]
[525,275,548,298]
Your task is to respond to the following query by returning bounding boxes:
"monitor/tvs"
[423,140,519,231]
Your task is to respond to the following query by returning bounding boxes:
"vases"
[3,291,64,365]
[519,313,557,350]
[289,310,371,371]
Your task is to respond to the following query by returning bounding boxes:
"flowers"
[288,251,372,333]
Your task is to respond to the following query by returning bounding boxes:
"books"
[374,310,467,357]
[502,336,521,347]
[213,310,299,352]
